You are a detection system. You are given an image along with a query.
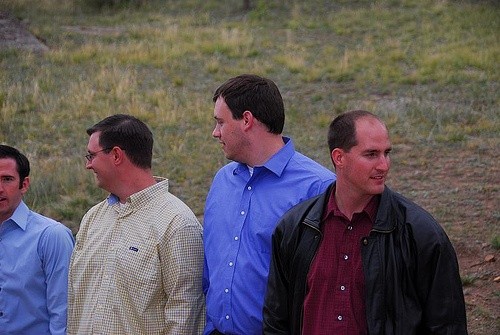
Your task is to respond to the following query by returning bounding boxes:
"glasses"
[85,147,113,162]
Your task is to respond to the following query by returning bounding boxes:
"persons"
[204,73,338,335]
[67,114,207,335]
[262,110,467,334]
[0,144,77,335]
